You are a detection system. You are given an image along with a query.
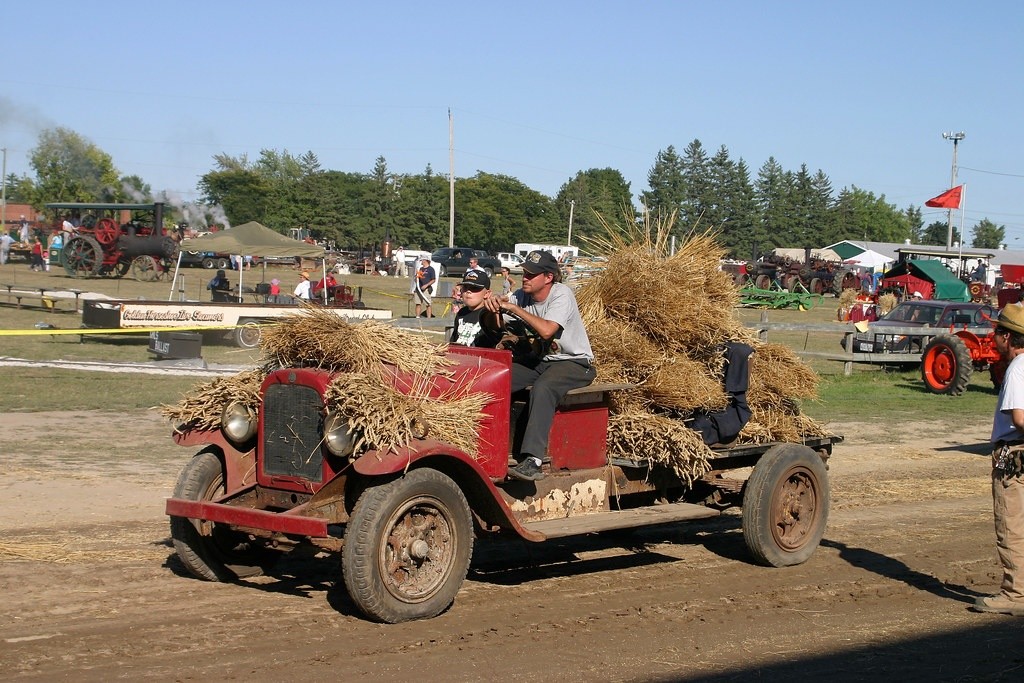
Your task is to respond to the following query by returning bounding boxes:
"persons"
[293,272,310,299]
[32,238,43,269]
[970,259,984,281]
[485,250,597,481]
[971,292,1024,616]
[230,254,238,270]
[19,215,29,249]
[0,231,15,264]
[50,230,62,248]
[314,268,338,295]
[63,214,81,230]
[206,270,225,290]
[394,246,409,278]
[414,257,436,318]
[466,257,485,272]
[862,270,871,290]
[501,267,516,295]
[271,279,281,294]
[452,282,464,317]
[243,255,252,271]
[449,270,504,349]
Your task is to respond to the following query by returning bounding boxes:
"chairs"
[210,284,233,302]
[309,280,322,300]
[916,312,929,321]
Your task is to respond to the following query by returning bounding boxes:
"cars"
[840,300,1001,370]
[558,256,609,275]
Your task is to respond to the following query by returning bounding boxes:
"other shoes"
[394,276,399,278]
[404,276,409,278]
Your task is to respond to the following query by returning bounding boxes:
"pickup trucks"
[431,247,501,280]
[496,250,527,276]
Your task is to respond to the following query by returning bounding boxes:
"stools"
[524,386,633,408]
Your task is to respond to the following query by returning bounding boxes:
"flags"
[925,185,962,209]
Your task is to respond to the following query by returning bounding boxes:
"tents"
[169,221,328,305]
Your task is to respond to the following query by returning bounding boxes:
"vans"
[456,249,488,259]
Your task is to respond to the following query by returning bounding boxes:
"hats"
[327,268,337,274]
[456,270,490,290]
[420,255,430,260]
[990,301,1024,334]
[399,246,403,249]
[298,272,310,281]
[515,250,559,275]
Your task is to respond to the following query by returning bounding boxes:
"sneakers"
[973,594,1024,616]
[508,457,544,481]
[508,455,518,465]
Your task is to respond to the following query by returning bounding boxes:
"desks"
[215,290,268,303]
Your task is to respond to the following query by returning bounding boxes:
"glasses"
[994,328,1006,335]
[462,285,484,293]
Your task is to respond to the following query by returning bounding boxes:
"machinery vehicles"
[47,201,318,283]
[163,304,846,624]
[721,238,1024,325]
[306,280,365,309]
[920,305,1011,396]
